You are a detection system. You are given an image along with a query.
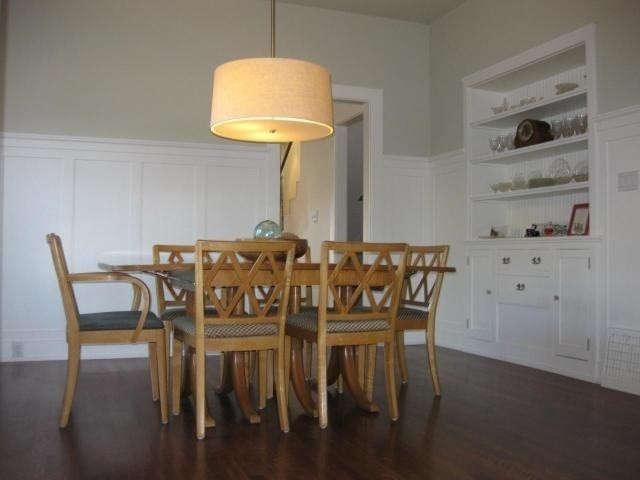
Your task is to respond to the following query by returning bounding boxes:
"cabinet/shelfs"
[458,21,602,386]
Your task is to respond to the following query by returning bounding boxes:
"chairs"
[285,239,408,430]
[356,244,452,403]
[174,238,296,439]
[150,243,233,399]
[46,232,172,430]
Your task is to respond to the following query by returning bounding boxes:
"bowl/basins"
[235,237,307,263]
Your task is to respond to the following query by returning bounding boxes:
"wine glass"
[488,134,515,155]
[550,112,587,141]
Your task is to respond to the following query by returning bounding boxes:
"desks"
[95,260,457,434]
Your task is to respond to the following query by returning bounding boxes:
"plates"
[572,162,587,182]
[545,158,571,187]
[554,82,578,92]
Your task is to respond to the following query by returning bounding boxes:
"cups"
[526,229,534,237]
[488,165,540,193]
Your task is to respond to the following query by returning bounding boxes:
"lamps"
[209,2,334,143]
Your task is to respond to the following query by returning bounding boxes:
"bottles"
[543,220,555,236]
